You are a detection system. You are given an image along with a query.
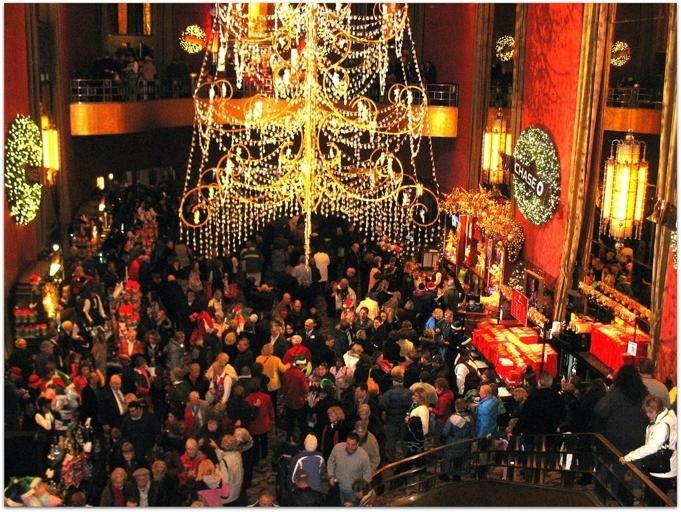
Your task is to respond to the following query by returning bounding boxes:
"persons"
[583,354,678,493]
[73,39,437,106]
[503,365,608,487]
[7,167,503,508]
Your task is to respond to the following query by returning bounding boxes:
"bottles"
[50,416,94,460]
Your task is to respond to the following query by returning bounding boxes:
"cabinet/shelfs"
[437,203,524,305]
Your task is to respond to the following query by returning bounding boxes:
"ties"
[116,390,125,411]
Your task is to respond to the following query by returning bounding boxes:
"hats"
[320,379,333,388]
[292,356,306,366]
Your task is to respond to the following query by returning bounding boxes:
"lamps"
[37,102,64,188]
[177,3,441,263]
[599,131,651,244]
[482,107,511,191]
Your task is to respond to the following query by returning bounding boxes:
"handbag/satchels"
[642,448,673,472]
